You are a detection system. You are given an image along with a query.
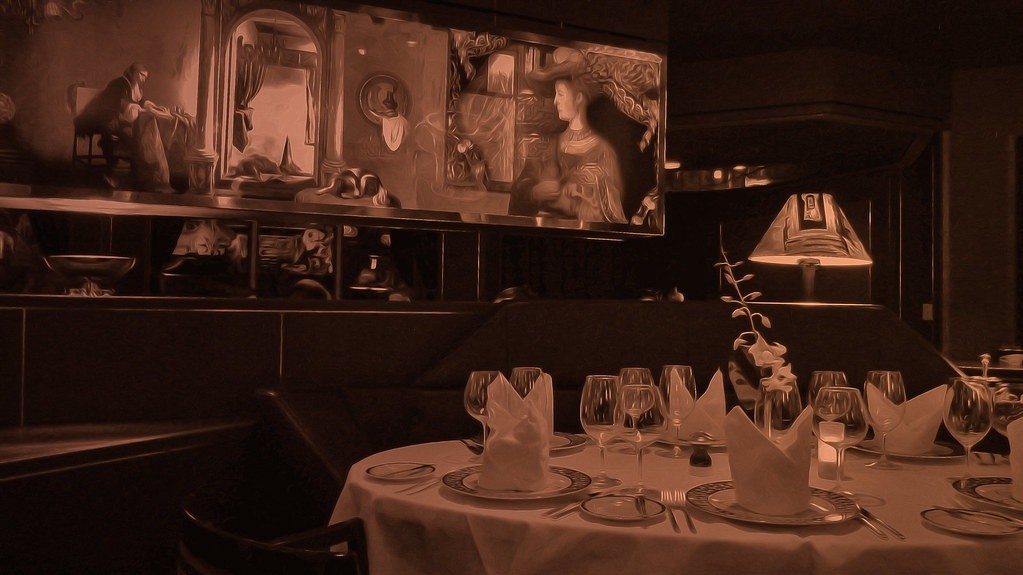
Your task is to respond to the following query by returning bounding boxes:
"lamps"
[748,193,874,303]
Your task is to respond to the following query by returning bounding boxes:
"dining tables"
[328,432,1023,575]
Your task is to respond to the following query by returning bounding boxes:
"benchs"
[254,297,1012,507]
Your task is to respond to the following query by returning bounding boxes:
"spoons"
[635,486,649,519]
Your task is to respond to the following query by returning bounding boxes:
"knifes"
[551,492,614,521]
[856,502,906,539]
[541,491,603,517]
[458,438,482,455]
[379,463,437,479]
[931,504,1023,528]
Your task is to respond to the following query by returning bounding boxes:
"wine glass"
[811,386,867,500]
[580,376,625,491]
[464,371,499,450]
[658,365,696,458]
[864,369,906,472]
[809,371,849,459]
[617,367,655,456]
[942,376,993,482]
[613,384,668,496]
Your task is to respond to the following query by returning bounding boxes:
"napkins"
[478,398,549,491]
[488,369,553,447]
[866,381,955,456]
[667,366,727,440]
[725,405,814,515]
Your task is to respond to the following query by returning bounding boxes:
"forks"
[392,472,448,493]
[675,488,696,533]
[661,490,681,533]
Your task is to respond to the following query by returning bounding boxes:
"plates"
[366,425,1022,534]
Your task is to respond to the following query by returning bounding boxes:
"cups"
[999,348,1023,369]
[510,367,541,401]
[993,383,1023,437]
[753,376,802,442]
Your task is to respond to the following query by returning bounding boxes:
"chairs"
[176,505,370,575]
